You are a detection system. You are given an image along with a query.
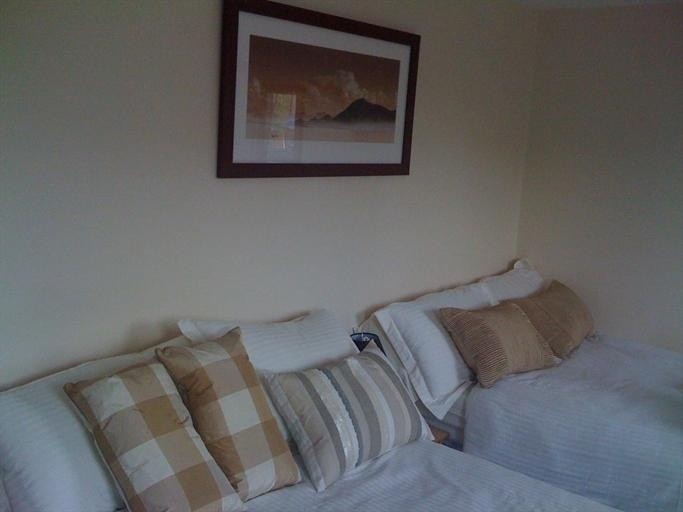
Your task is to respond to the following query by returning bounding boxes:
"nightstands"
[429,425,450,442]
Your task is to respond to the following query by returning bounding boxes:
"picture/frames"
[217,0,421,179]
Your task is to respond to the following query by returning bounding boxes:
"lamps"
[350,332,385,355]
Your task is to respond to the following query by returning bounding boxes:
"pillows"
[440,301,562,388]
[1,333,195,512]
[154,326,302,501]
[178,309,360,374]
[374,281,501,420]
[63,358,245,512]
[479,258,544,305]
[498,280,594,359]
[352,315,420,403]
[261,340,434,493]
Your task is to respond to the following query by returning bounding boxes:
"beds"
[352,258,683,512]
[1,308,621,512]
